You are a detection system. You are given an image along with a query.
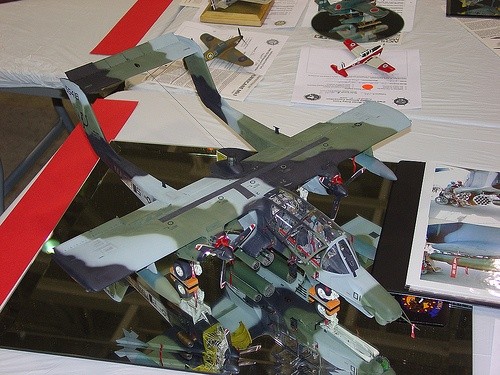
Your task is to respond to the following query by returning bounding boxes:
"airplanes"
[200,27,254,67]
[314,0,389,24]
[210,0,272,11]
[442,165,500,205]
[330,39,396,78]
[329,20,388,41]
[44,51,414,375]
